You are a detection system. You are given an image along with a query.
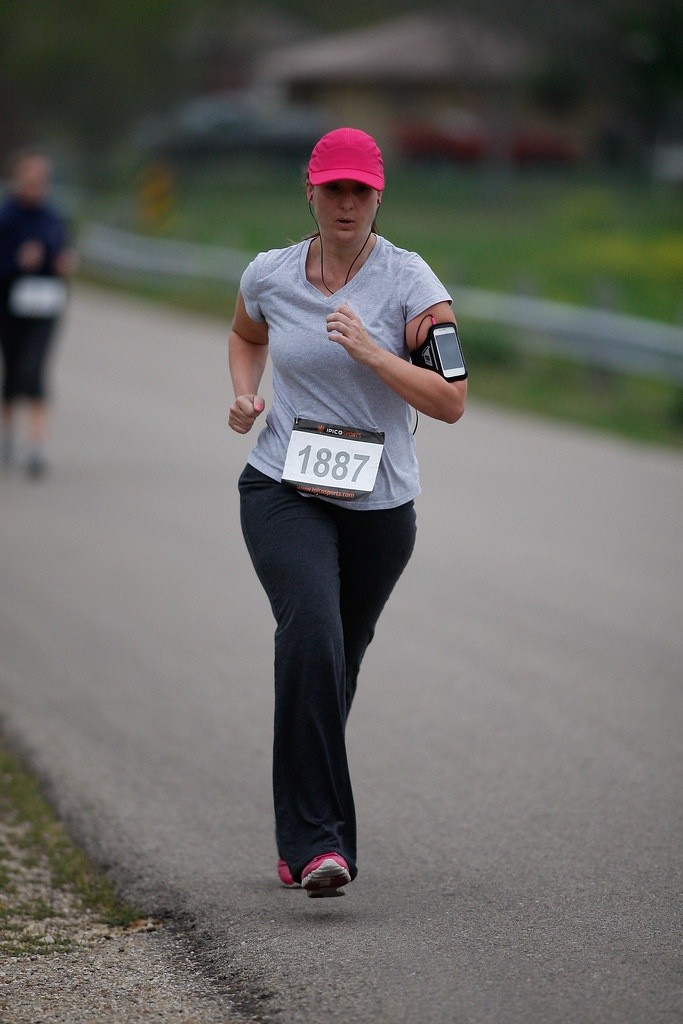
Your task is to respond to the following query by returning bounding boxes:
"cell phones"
[431,325,467,381]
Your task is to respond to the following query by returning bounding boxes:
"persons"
[227,125,470,899]
[0,148,80,480]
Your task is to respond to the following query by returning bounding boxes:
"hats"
[306,127,384,194]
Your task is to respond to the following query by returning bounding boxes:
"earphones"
[376,194,382,204]
[307,191,315,201]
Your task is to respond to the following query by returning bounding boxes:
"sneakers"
[276,850,300,890]
[296,849,351,903]
